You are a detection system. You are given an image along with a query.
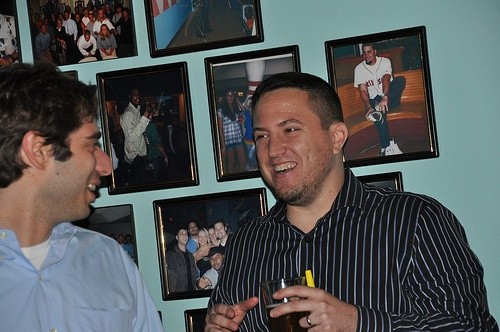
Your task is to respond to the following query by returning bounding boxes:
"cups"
[261,276,309,332]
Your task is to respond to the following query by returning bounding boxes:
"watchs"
[381,93,388,97]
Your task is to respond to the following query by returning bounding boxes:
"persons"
[194,0,213,38]
[353,43,406,156]
[0,14,19,68]
[218,88,260,172]
[110,233,134,258]
[1,62,163,332]
[204,72,500,332]
[166,219,232,293]
[34,0,133,66]
[118,88,171,179]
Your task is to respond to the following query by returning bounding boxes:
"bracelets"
[10,55,15,62]
[367,105,371,109]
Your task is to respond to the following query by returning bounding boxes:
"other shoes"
[368,111,383,122]
[387,139,402,155]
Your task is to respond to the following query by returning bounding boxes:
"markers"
[305,269,316,288]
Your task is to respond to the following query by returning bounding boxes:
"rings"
[306,316,311,325]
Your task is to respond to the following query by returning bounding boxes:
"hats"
[205,247,223,257]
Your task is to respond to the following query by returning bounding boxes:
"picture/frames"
[142,0,262,58]
[26,1,135,70]
[153,188,274,304]
[324,24,439,192]
[81,202,140,267]
[0,0,23,67]
[94,62,199,197]
[184,307,210,332]
[204,43,301,184]
